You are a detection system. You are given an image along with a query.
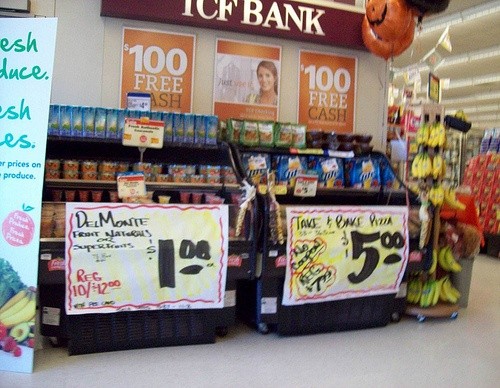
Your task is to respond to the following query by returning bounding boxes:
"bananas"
[453,109,468,122]
[0,286,37,327]
[428,182,466,210]
[412,123,448,179]
[405,244,462,308]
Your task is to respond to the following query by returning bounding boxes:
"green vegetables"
[0,258,25,306]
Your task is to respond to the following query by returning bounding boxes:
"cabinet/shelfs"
[36,102,261,357]
[221,112,481,334]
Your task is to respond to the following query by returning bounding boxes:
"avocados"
[9,323,31,344]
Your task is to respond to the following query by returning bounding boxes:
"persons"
[252,61,279,108]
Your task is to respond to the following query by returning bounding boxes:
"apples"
[0,323,21,356]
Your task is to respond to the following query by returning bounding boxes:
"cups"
[109,191,119,202]
[54,190,62,201]
[147,191,154,201]
[231,193,242,203]
[79,190,89,202]
[180,192,191,204]
[192,192,203,204]
[205,193,216,204]
[92,191,102,202]
[159,196,171,204]
[64,190,76,202]
